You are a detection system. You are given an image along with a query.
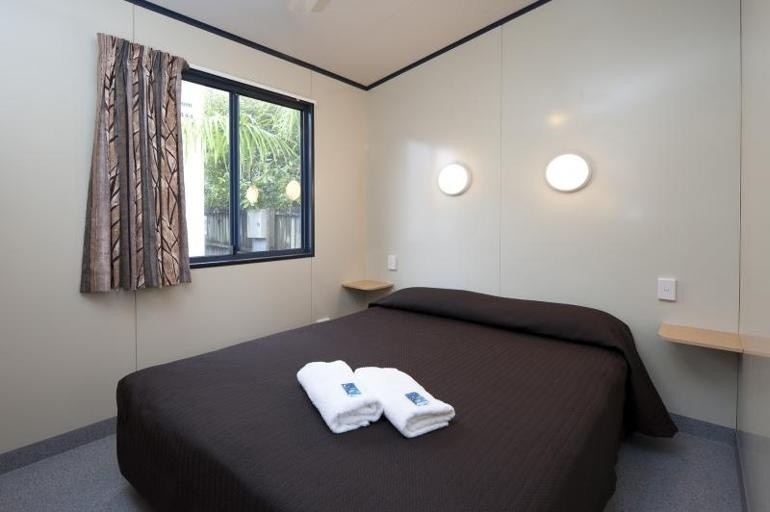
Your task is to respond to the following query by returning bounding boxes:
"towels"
[296,357,383,433]
[353,364,454,439]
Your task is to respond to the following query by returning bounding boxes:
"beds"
[117,287,679,512]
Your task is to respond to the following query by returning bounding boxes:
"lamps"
[545,153,592,192]
[437,163,472,195]
